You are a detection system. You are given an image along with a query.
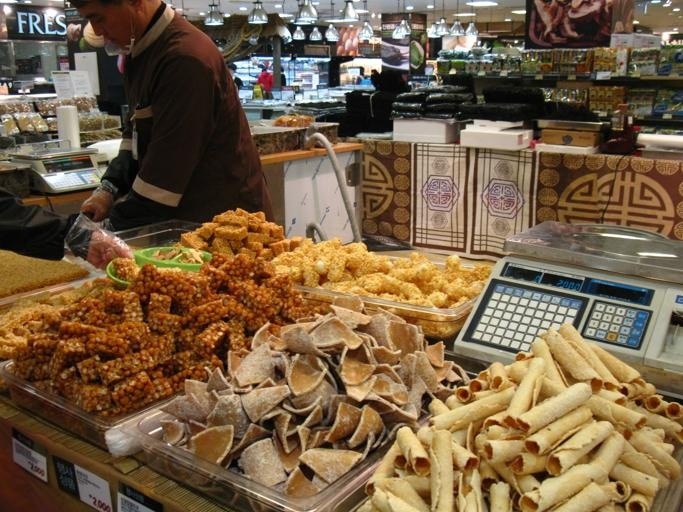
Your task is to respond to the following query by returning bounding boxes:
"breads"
[275,115,312,127]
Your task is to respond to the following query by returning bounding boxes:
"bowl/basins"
[133,246,212,272]
[106,257,132,286]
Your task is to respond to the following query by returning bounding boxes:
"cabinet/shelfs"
[517,48,683,136]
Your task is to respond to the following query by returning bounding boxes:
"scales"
[450,221,683,399]
[9,146,101,194]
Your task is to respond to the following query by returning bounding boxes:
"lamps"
[293,26,306,41]
[465,1,479,35]
[393,3,413,40]
[359,1,373,41]
[292,0,319,26]
[449,0,465,36]
[338,0,359,22]
[247,0,268,24]
[325,23,341,42]
[309,26,323,41]
[427,1,441,39]
[436,1,449,35]
[204,1,225,26]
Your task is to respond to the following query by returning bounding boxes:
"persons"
[257,69,272,91]
[228,63,242,94]
[0,184,134,271]
[278,66,286,89]
[65,0,275,232]
